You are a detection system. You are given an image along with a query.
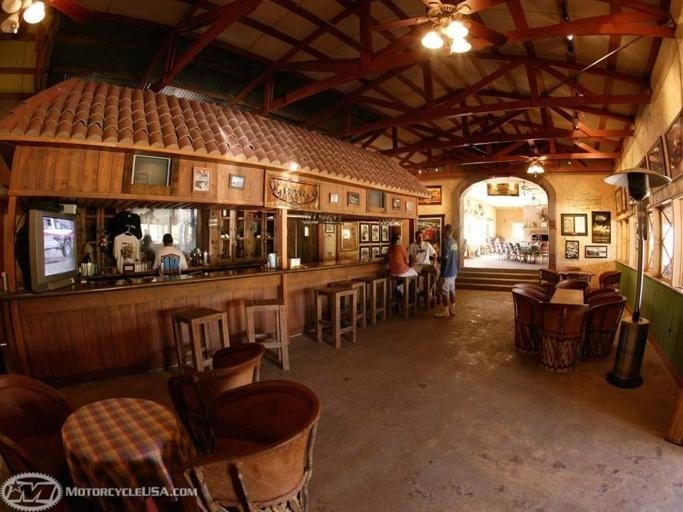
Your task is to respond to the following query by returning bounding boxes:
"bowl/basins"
[109,276,157,286]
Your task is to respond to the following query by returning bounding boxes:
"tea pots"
[266,252,281,269]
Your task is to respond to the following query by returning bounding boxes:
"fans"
[365,2,506,61]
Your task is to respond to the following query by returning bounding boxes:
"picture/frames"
[346,191,360,208]
[607,112,681,211]
[226,173,245,191]
[325,212,445,260]
[192,165,210,195]
[328,192,340,204]
[392,197,401,210]
[405,201,413,213]
[559,207,613,263]
[416,185,443,205]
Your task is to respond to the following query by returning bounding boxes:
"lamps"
[602,167,671,385]
[0,1,50,43]
[525,160,546,179]
[418,22,473,56]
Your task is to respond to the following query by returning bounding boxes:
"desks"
[60,397,182,511]
[556,269,595,284]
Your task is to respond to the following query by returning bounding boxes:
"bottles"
[188,248,208,265]
[160,256,164,272]
[76,254,97,276]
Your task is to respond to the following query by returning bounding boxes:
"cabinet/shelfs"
[200,207,266,266]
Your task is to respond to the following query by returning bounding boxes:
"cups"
[259,259,270,273]
[133,262,148,273]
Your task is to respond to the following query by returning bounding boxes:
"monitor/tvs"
[17,210,77,293]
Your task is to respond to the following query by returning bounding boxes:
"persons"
[435,224,459,317]
[382,235,419,297]
[152,234,188,276]
[406,231,441,297]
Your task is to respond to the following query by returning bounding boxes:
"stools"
[244,297,290,370]
[395,275,417,318]
[170,307,232,373]
[328,281,366,328]
[417,271,438,307]
[314,284,357,349]
[353,275,384,321]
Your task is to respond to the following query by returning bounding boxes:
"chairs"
[167,343,267,453]
[462,230,547,265]
[534,265,557,284]
[0,375,73,511]
[597,269,621,289]
[507,280,621,373]
[186,376,320,511]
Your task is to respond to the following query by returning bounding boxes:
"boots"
[449,303,456,315]
[435,306,450,317]
